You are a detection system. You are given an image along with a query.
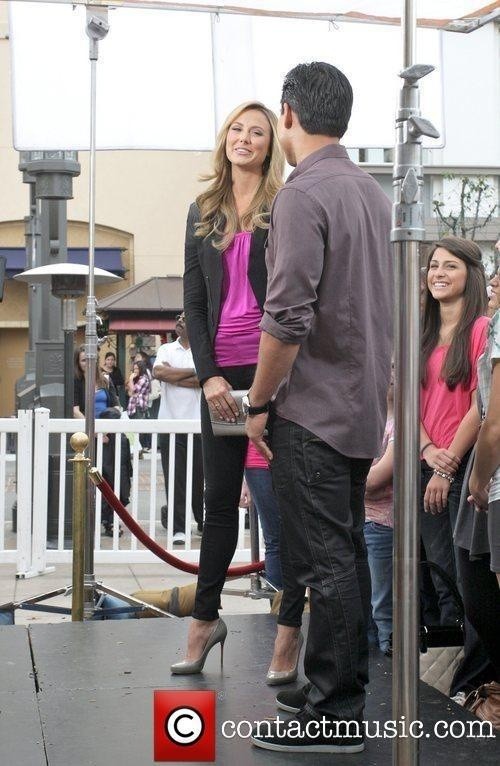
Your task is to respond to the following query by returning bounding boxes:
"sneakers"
[103,521,124,539]
[275,683,311,713]
[160,505,169,529]
[172,530,187,545]
[250,714,364,754]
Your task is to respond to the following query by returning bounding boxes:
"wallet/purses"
[210,389,272,438]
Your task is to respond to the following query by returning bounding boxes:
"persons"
[238,60,392,754]
[72,233,500,736]
[170,100,308,688]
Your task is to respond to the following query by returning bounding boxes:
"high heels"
[266,631,305,686]
[171,619,227,675]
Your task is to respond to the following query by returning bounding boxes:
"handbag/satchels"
[463,681,500,729]
[385,557,468,702]
